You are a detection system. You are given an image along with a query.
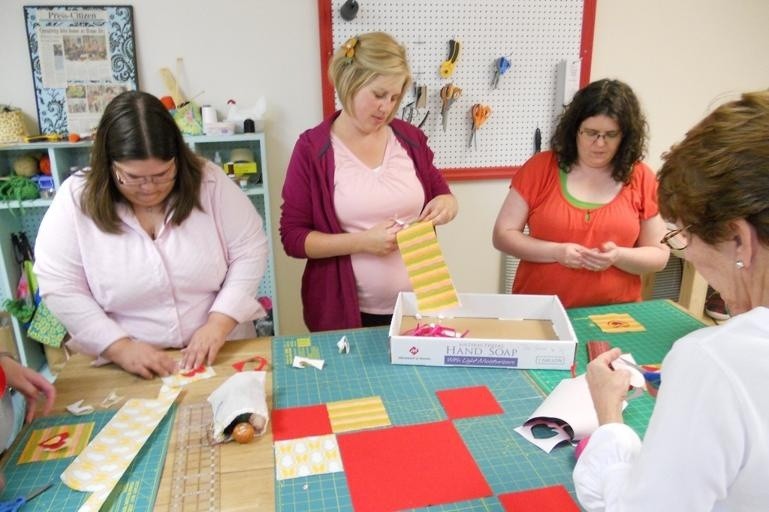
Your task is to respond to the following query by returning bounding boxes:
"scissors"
[495,56,510,89]
[470,104,491,149]
[441,85,463,131]
[390,217,420,236]
[618,355,660,390]
[1,480,54,511]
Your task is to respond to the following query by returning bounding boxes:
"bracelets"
[0,352,15,361]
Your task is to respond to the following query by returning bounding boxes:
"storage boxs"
[386,291,581,370]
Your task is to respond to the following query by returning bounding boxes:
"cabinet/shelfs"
[0,130,278,374]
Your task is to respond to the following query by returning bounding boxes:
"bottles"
[213,150,224,172]
[70,166,79,177]
[243,117,255,132]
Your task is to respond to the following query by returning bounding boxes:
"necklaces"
[135,204,160,213]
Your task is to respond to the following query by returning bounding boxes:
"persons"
[572,90,768,512]
[33,90,270,382]
[0,344,53,424]
[492,80,671,311]
[278,31,458,333]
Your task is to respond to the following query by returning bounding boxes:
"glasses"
[110,156,178,186]
[577,128,623,143]
[660,223,694,250]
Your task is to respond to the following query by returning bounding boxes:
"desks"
[1,298,723,512]
